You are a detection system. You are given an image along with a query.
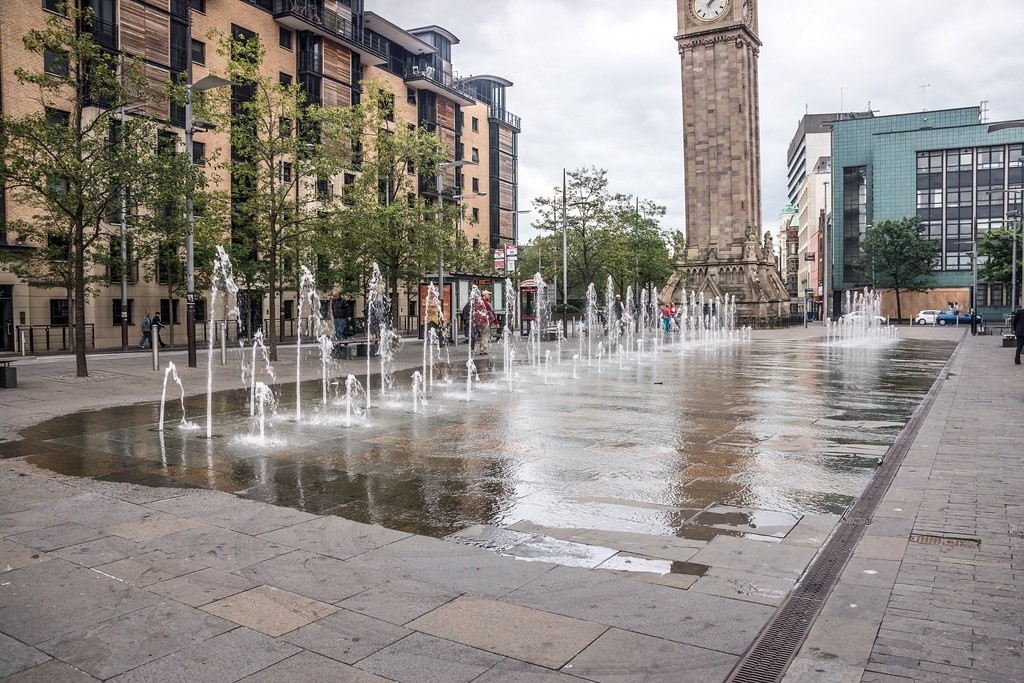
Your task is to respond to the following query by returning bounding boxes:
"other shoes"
[162,344,167,348]
[150,346,153,349]
[138,345,144,348]
[480,352,488,355]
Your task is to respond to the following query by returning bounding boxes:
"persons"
[947,302,960,309]
[136,311,167,349]
[344,316,357,339]
[1012,309,1024,366]
[581,294,716,343]
[462,290,500,355]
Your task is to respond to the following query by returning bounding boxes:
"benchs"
[534,325,565,341]
[1003,334,1017,347]
[984,326,1012,334]
[333,339,380,359]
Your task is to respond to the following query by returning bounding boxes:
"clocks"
[741,0,753,27]
[687,0,732,26]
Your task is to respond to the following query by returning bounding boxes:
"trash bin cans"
[0,359,18,388]
[497,314,514,341]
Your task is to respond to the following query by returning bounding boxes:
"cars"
[915,309,983,326]
[838,311,887,325]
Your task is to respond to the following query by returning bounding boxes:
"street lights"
[954,241,978,335]
[183,76,238,369]
[278,143,316,342]
[563,201,598,339]
[435,160,479,348]
[111,102,146,351]
[1003,209,1019,347]
[823,181,830,327]
[451,192,487,343]
[507,210,531,340]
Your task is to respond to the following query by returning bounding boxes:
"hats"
[482,290,492,294]
[155,312,161,314]
